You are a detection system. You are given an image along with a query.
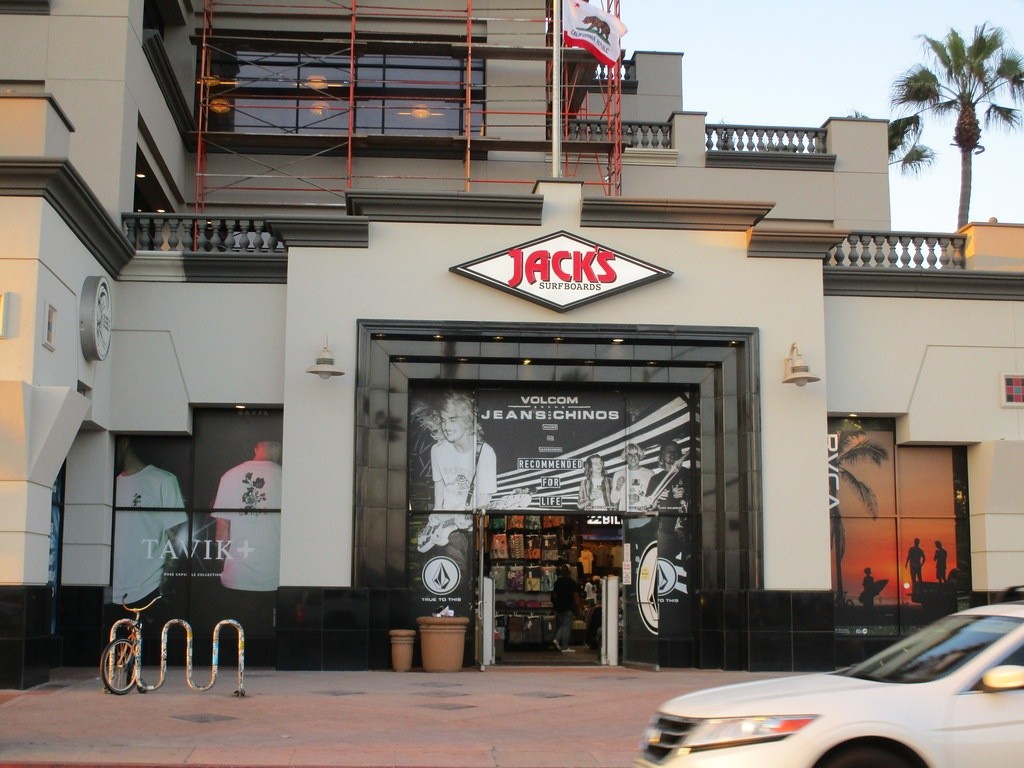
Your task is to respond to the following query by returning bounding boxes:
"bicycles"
[100,593,163,695]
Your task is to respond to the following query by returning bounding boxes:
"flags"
[564,0,627,69]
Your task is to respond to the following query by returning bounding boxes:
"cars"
[634,600,1024,768]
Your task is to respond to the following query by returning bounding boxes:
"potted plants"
[415,598,470,671]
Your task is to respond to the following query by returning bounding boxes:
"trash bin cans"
[417,616,469,671]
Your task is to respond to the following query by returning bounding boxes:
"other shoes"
[562,648,574,652]
[553,640,561,650]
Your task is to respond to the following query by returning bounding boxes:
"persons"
[411,390,498,611]
[210,437,282,601]
[552,564,580,652]
[114,441,189,627]
[578,439,690,559]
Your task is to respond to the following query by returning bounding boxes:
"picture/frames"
[42,299,59,352]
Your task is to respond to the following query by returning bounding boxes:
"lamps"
[780,341,820,387]
[304,334,345,379]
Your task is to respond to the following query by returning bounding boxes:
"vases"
[389,629,416,671]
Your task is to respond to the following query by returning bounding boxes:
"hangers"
[616,543,622,548]
[543,611,555,620]
[598,542,608,550]
[495,611,503,618]
[526,612,540,620]
[509,612,523,618]
